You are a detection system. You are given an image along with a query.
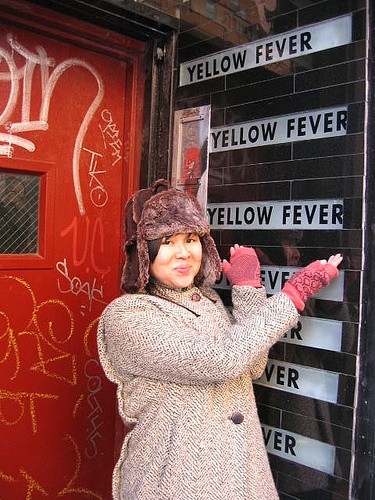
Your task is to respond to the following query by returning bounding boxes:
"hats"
[120,179,221,295]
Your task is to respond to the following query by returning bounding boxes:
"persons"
[96,181,343,500]
